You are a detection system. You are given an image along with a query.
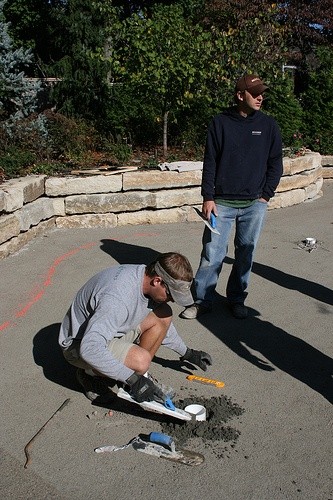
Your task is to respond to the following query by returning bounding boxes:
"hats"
[155,261,194,306]
[236,74,270,96]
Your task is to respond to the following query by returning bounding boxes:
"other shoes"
[182,303,213,319]
[232,303,248,318]
[80,375,118,405]
[147,371,175,399]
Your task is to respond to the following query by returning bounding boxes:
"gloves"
[126,372,163,403]
[184,346,212,372]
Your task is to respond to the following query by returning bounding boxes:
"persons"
[182,75,284,319]
[58,252,212,403]
